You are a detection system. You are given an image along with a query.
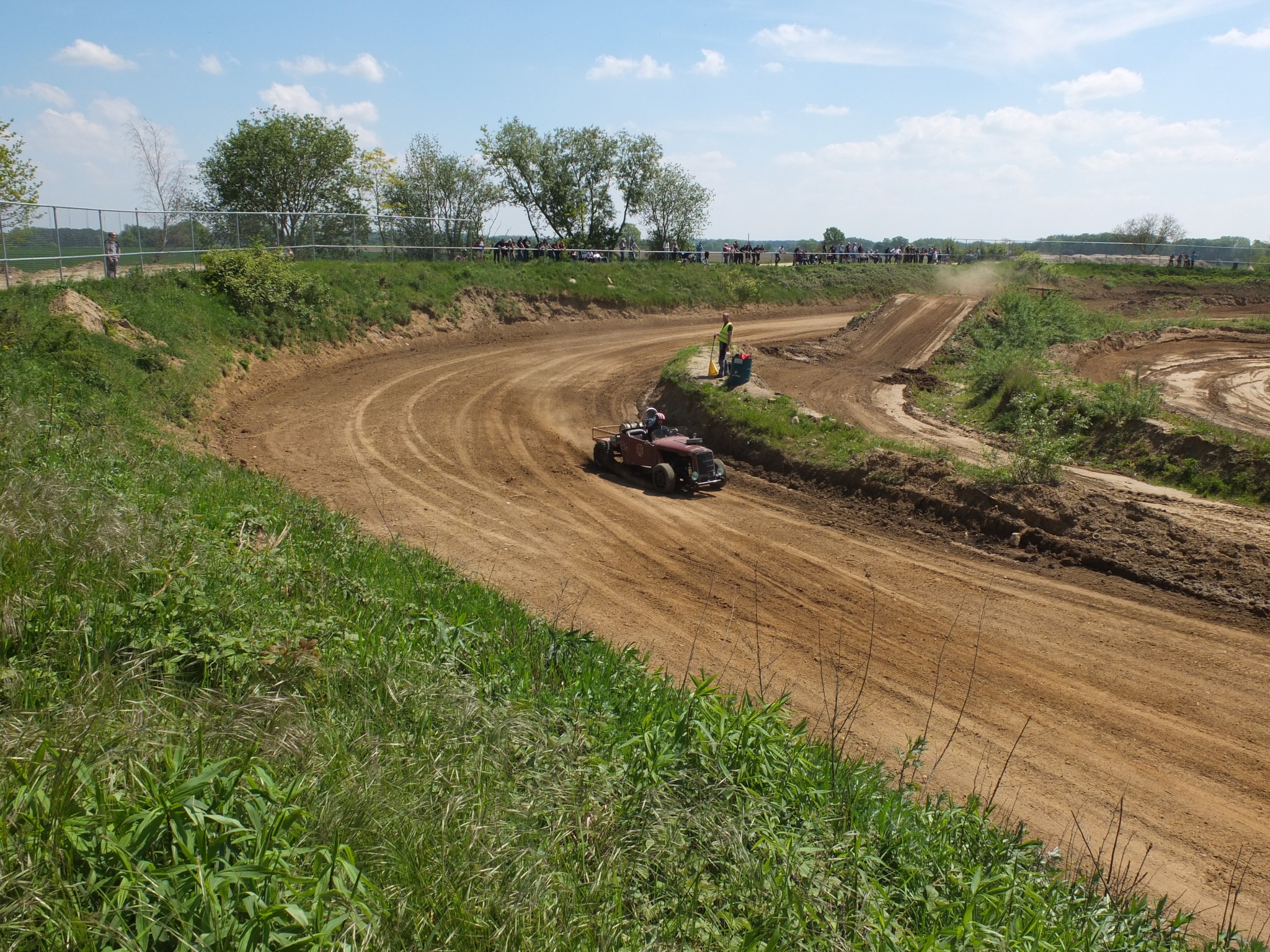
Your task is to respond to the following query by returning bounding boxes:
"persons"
[454,235,641,264]
[714,313,734,378]
[1169,251,1198,266]
[104,232,122,279]
[792,241,951,266]
[663,238,709,265]
[723,241,767,266]
[647,413,678,443]
[1247,263,1254,270]
[775,245,786,266]
[268,241,294,263]
[956,247,981,265]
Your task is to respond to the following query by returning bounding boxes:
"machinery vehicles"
[592,407,727,495]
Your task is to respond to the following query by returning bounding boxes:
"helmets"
[654,413,665,424]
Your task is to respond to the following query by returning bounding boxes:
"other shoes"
[715,374,724,378]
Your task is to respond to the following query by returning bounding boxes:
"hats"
[621,238,626,242]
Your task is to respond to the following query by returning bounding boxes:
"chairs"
[581,250,590,262]
[679,255,688,264]
[626,428,647,440]
[568,249,578,262]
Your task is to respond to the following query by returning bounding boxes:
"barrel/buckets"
[729,356,752,387]
